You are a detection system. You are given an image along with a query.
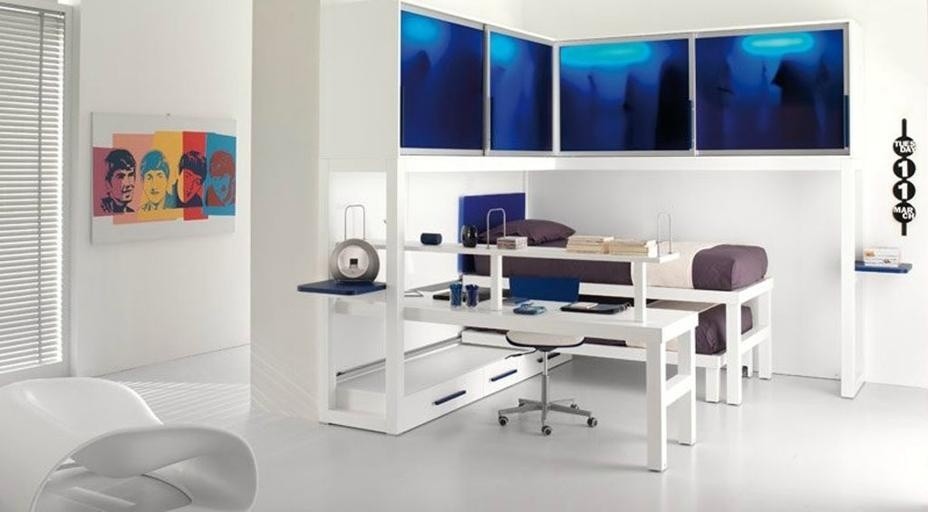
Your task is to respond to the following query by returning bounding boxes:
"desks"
[333,291,699,471]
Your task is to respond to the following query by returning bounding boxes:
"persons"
[168,147,209,209]
[205,150,236,208]
[99,147,137,212]
[139,147,179,211]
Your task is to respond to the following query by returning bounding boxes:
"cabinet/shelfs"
[397,1,860,169]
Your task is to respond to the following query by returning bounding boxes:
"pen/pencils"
[449,284,462,305]
[466,285,477,305]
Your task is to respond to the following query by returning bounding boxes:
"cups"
[449,290,479,310]
[461,224,478,247]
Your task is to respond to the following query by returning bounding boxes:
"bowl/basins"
[421,233,442,245]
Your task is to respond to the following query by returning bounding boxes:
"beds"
[458,193,773,405]
[462,295,753,403]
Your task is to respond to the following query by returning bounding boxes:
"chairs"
[0,378,259,512]
[498,276,598,435]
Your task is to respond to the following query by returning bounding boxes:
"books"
[562,234,660,259]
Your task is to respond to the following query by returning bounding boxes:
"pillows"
[477,219,575,244]
